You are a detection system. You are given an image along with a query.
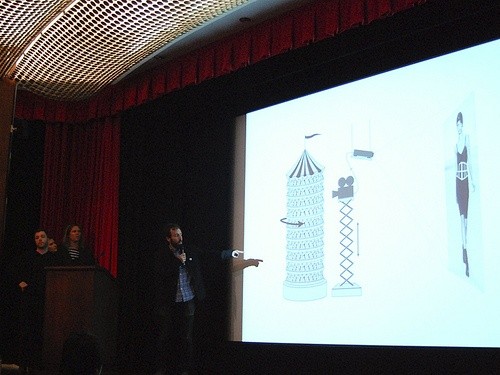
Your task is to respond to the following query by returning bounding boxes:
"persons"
[18,231,52,375]
[54,224,96,267]
[46,237,58,251]
[151,223,244,374]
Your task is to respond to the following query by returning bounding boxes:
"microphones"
[180,244,186,266]
